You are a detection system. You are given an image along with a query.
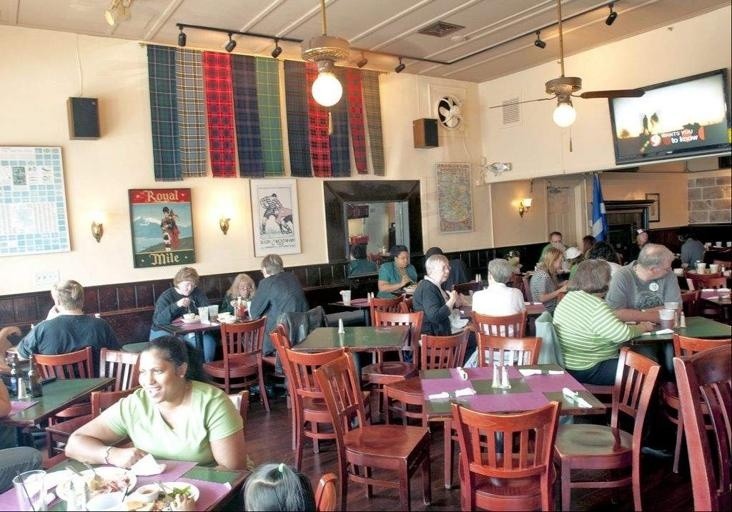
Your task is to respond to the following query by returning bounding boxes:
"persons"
[250,255,309,357]
[0,379,42,497]
[219,273,256,315]
[0,324,23,373]
[650,113,659,126]
[348,245,376,275]
[378,245,473,361]
[15,280,120,377]
[161,207,173,251]
[530,230,622,315]
[551,257,671,457]
[169,463,306,511]
[259,193,283,234]
[605,228,704,367]
[149,267,216,363]
[64,335,248,470]
[643,115,650,134]
[274,206,298,232]
[472,259,526,366]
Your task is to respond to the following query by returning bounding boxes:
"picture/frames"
[645,193,660,222]
[128,188,195,268]
[249,178,302,257]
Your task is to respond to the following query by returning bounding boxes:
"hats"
[565,248,584,259]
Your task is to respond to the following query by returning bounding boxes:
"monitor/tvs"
[607,66,732,166]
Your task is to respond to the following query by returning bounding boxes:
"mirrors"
[323,180,424,279]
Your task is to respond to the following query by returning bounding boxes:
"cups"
[341,290,351,306]
[197,304,219,323]
[659,302,679,330]
[11,469,48,511]
[694,241,732,274]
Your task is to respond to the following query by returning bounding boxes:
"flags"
[592,173,609,242]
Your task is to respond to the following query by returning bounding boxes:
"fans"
[488,88,645,109]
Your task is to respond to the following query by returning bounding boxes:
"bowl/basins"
[135,484,159,503]
[183,313,195,320]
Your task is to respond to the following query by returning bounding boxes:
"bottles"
[476,273,482,283]
[680,311,686,328]
[490,364,512,389]
[337,318,345,334]
[720,264,725,277]
[236,296,245,322]
[367,292,375,304]
[10,352,43,403]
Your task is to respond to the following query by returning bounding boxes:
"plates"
[713,245,723,248]
[56,466,137,502]
[216,316,240,324]
[180,315,200,323]
[403,287,416,292]
[123,481,200,511]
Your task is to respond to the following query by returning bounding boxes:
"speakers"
[66,96,102,143]
[411,117,441,150]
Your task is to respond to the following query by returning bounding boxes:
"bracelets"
[105,445,112,464]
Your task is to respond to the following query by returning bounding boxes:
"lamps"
[104,0,132,25]
[530,2,618,48]
[176,23,405,73]
[300,0,349,108]
[545,0,582,127]
[519,198,532,218]
